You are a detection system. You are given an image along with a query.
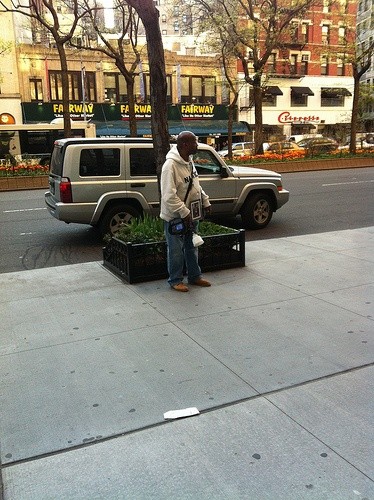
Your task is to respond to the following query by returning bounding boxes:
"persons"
[158,130,211,291]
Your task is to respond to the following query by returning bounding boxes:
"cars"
[213,131,374,161]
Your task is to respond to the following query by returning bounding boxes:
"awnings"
[320,87,352,97]
[95,118,249,140]
[290,87,314,96]
[262,86,283,95]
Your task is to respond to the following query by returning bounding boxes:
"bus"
[0,124,98,169]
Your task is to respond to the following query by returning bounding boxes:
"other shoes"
[173,284,188,292]
[191,277,211,287]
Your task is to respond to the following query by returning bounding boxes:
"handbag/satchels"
[169,218,185,235]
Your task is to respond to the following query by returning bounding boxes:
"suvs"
[44,133,290,243]
